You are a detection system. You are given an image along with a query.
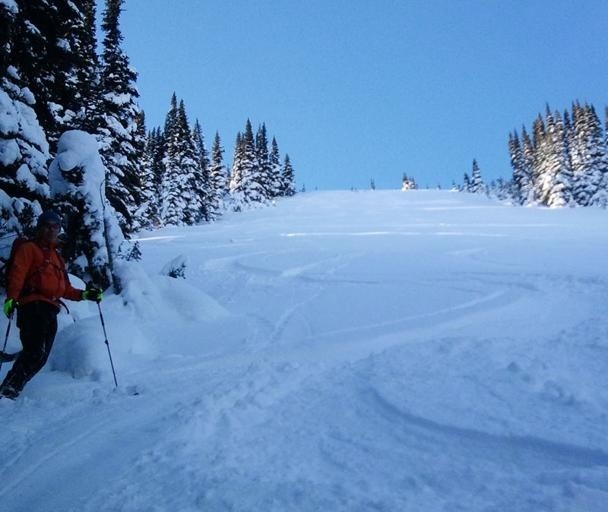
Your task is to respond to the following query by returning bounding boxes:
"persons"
[1,213,102,400]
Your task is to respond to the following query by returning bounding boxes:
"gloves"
[3,297,18,319]
[81,288,102,304]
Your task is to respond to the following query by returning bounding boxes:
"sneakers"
[0,379,20,398]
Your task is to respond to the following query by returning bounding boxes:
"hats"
[37,210,62,233]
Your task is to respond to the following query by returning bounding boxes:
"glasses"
[41,222,60,229]
